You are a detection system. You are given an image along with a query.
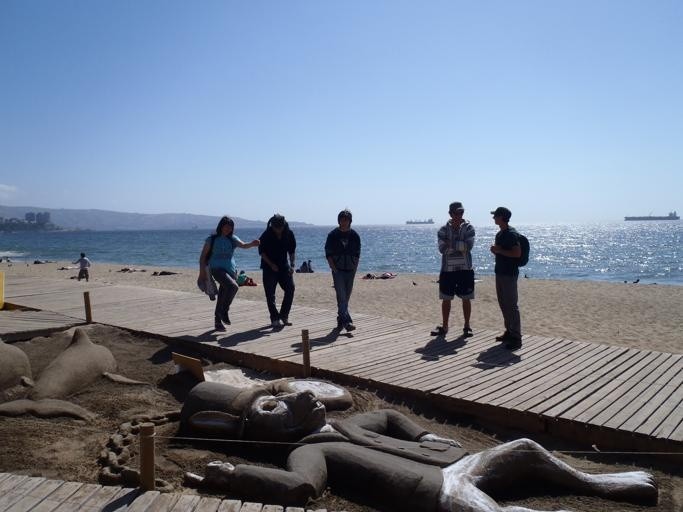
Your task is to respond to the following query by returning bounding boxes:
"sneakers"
[272,314,291,327]
[215,311,230,330]
[337,316,355,331]
[496,331,521,349]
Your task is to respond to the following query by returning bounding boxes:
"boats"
[405,218,435,225]
[624,210,681,221]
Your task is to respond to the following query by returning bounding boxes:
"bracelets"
[291,263,295,267]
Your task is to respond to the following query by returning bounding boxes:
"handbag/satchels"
[504,232,529,266]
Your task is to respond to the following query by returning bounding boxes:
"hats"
[450,202,463,212]
[491,207,511,218]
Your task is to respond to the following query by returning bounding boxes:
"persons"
[490,207,522,350]
[200,216,261,332]
[367,273,377,279]
[296,259,314,273]
[0,257,57,264]
[72,252,92,282]
[430,201,476,337]
[258,212,296,332]
[236,270,257,286]
[380,272,392,279]
[57,266,79,270]
[121,268,146,273]
[324,209,361,332]
[152,271,178,276]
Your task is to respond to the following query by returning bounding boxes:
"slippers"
[463,328,473,337]
[431,327,447,336]
